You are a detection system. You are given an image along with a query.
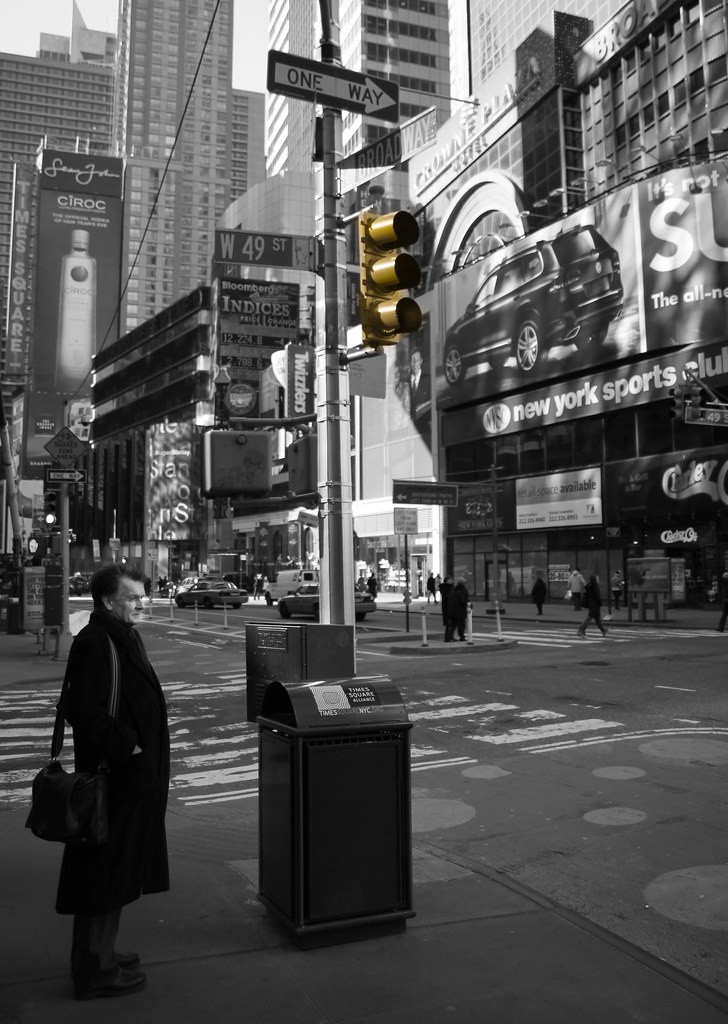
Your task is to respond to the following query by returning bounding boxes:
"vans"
[264,568,320,606]
[176,576,227,596]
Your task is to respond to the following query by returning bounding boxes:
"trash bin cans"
[7,597,25,635]
[255,675,417,950]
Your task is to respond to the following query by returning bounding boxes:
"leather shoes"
[73,969,147,1001]
[106,951,140,970]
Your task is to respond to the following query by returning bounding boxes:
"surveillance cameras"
[80,414,91,426]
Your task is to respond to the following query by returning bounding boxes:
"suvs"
[441,223,626,388]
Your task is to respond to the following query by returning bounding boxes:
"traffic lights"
[43,491,57,527]
[689,384,706,419]
[358,210,423,346]
[27,536,48,558]
[670,386,685,421]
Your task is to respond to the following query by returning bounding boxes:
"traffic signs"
[46,468,87,484]
[266,49,401,124]
[392,478,459,508]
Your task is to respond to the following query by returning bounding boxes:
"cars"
[175,580,249,609]
[159,579,181,598]
[69,574,94,596]
[277,582,377,623]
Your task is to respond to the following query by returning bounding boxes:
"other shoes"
[445,636,457,643]
[602,628,611,635]
[459,636,466,641]
[576,630,586,637]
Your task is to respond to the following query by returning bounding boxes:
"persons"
[611,571,625,610]
[530,577,547,615]
[577,574,610,639]
[427,573,441,604]
[367,572,378,601]
[567,568,587,611]
[55,562,170,999]
[383,346,432,440]
[144,578,152,596]
[254,574,263,600]
[156,576,167,592]
[504,569,517,597]
[714,571,728,633]
[439,575,470,642]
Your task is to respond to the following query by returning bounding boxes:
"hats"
[458,578,465,583]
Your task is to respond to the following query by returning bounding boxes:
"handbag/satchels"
[23,759,106,847]
[564,590,571,601]
[580,593,589,608]
